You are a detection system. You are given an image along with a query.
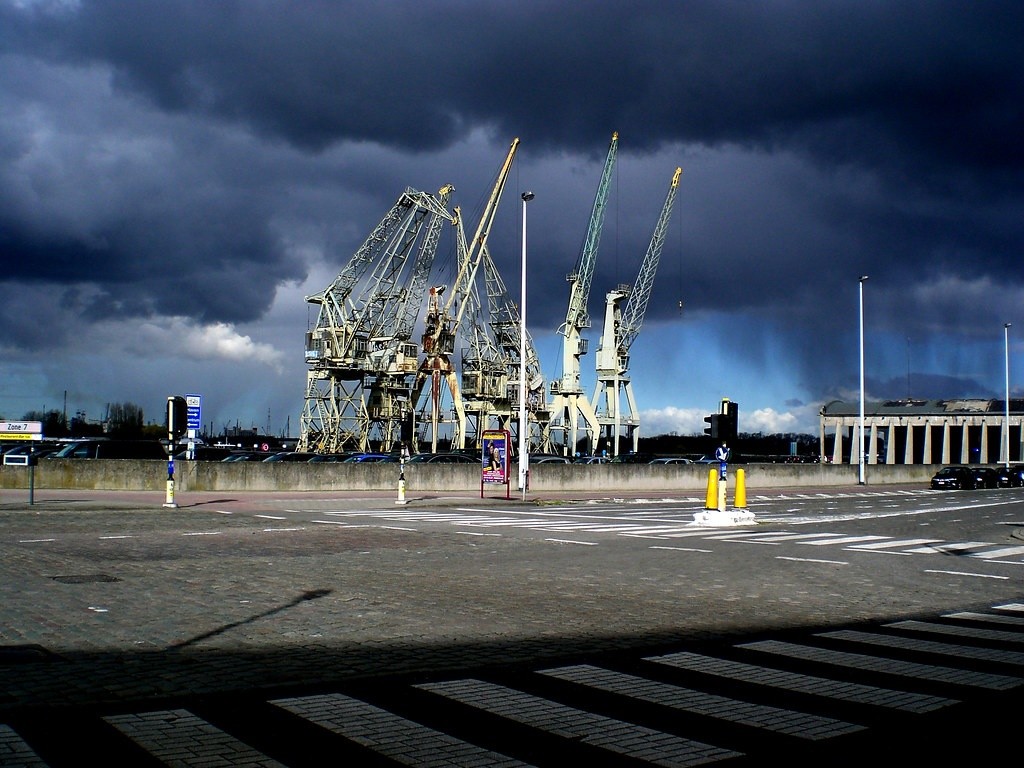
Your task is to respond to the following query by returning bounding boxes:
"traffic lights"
[703,414,718,438]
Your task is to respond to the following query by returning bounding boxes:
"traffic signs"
[185,394,201,430]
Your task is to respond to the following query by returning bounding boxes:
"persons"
[486,439,503,471]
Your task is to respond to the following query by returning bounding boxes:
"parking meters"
[162,395,189,507]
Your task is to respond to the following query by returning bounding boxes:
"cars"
[649,459,690,464]
[220,452,394,463]
[530,457,571,464]
[0,439,107,466]
[971,467,996,490]
[995,465,1024,488]
[930,466,972,490]
[573,457,611,465]
[691,460,728,465]
[404,453,481,464]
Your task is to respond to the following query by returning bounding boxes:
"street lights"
[1004,322,1011,470]
[859,274,869,485]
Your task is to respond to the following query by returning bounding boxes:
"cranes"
[581,166,683,459]
[295,138,560,456]
[538,129,620,459]
